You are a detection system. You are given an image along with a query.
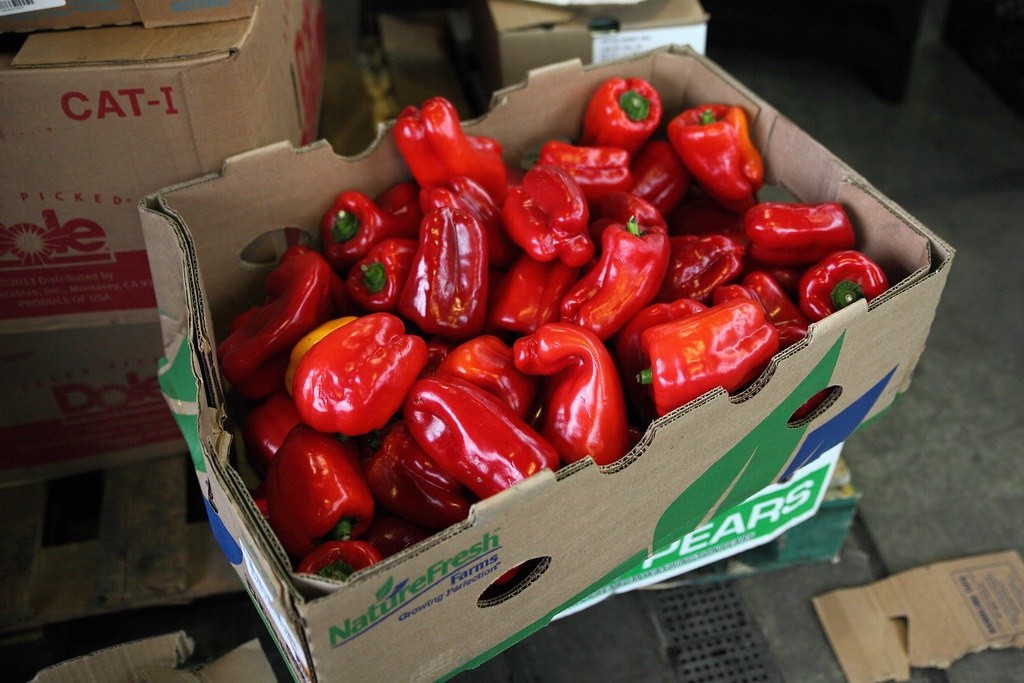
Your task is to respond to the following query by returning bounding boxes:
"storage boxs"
[0,0,956,683]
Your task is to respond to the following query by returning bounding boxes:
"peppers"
[215,76,888,598]
